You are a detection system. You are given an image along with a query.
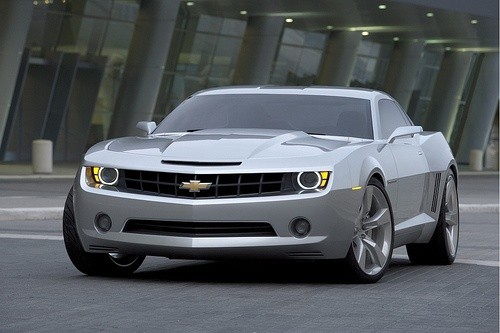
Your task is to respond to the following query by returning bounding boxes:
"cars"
[59,83,462,286]
